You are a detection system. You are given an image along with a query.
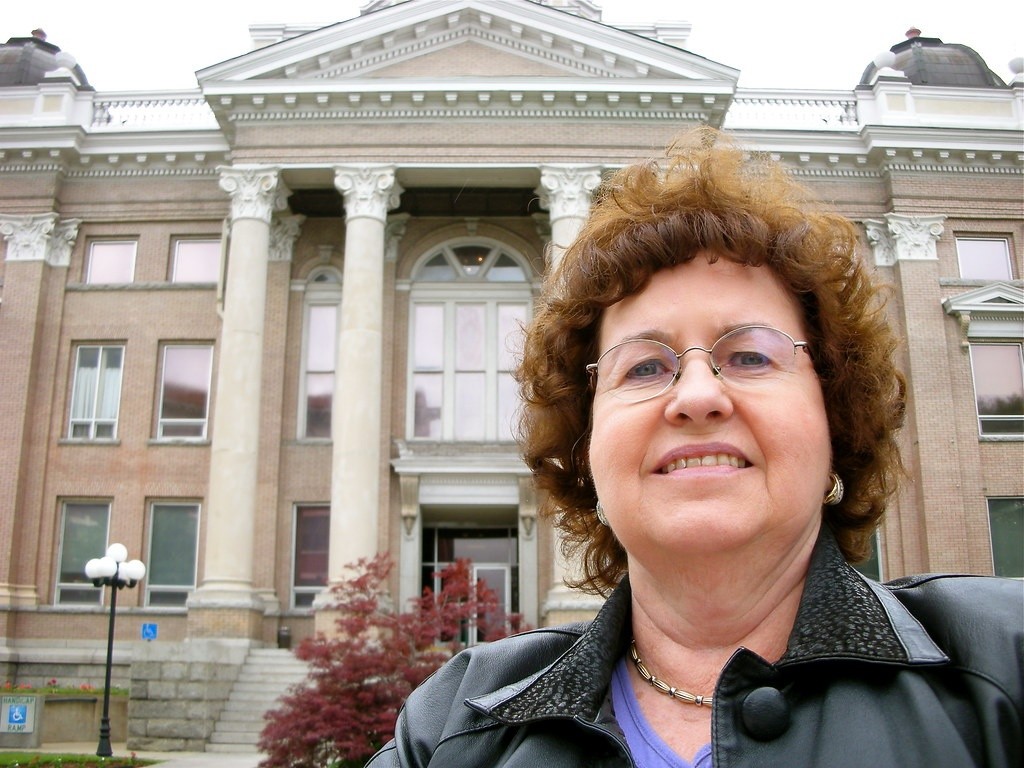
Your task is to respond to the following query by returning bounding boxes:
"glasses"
[586,325,814,404]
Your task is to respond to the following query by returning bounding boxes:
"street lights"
[84,542,147,758]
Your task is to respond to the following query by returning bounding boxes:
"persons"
[359,127,1024,768]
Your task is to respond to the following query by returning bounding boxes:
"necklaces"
[629,637,713,707]
